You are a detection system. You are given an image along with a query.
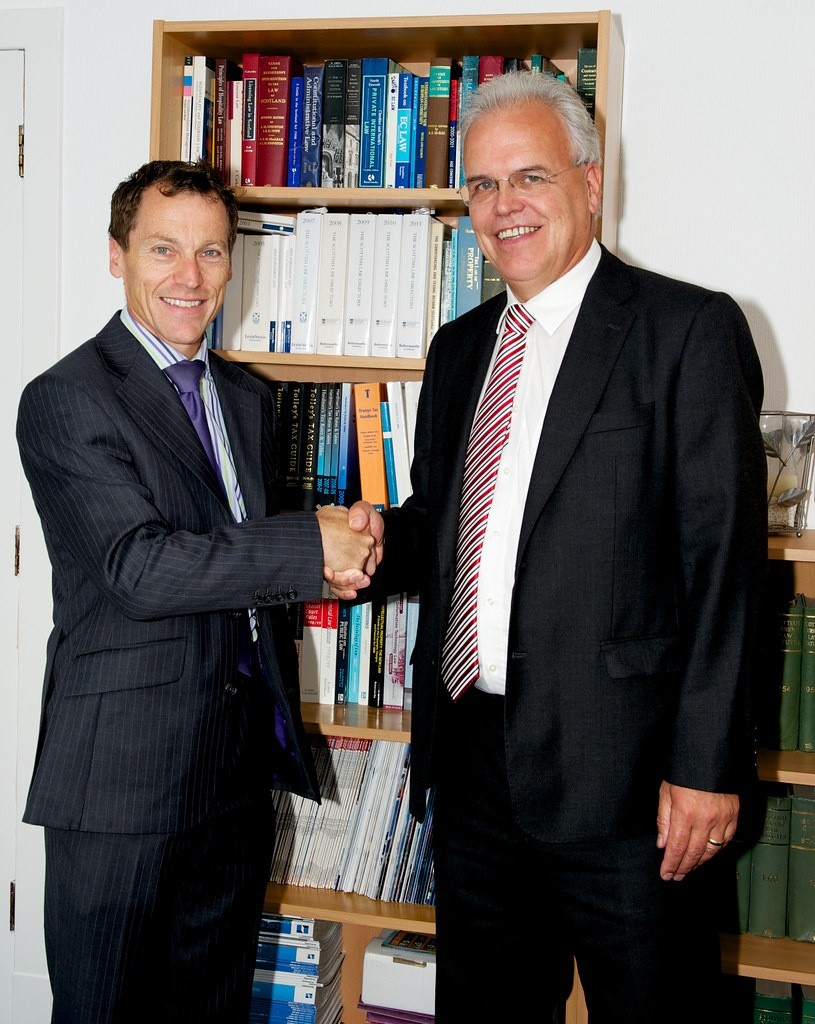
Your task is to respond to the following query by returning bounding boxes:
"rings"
[708,838,726,845]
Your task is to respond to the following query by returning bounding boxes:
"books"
[753,592,814,750]
[205,209,506,356]
[291,594,419,711]
[265,735,437,907]
[262,379,421,512]
[251,911,345,1023]
[752,979,814,1022]
[716,784,815,942]
[178,47,598,190]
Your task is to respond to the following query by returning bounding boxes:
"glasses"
[456,160,588,208]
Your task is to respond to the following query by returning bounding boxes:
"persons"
[19,155,376,1023]
[325,70,768,1024]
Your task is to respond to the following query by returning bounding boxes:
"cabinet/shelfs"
[148,10,623,1024]
[735,535,815,1024]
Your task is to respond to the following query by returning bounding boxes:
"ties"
[164,359,293,758]
[440,304,534,704]
